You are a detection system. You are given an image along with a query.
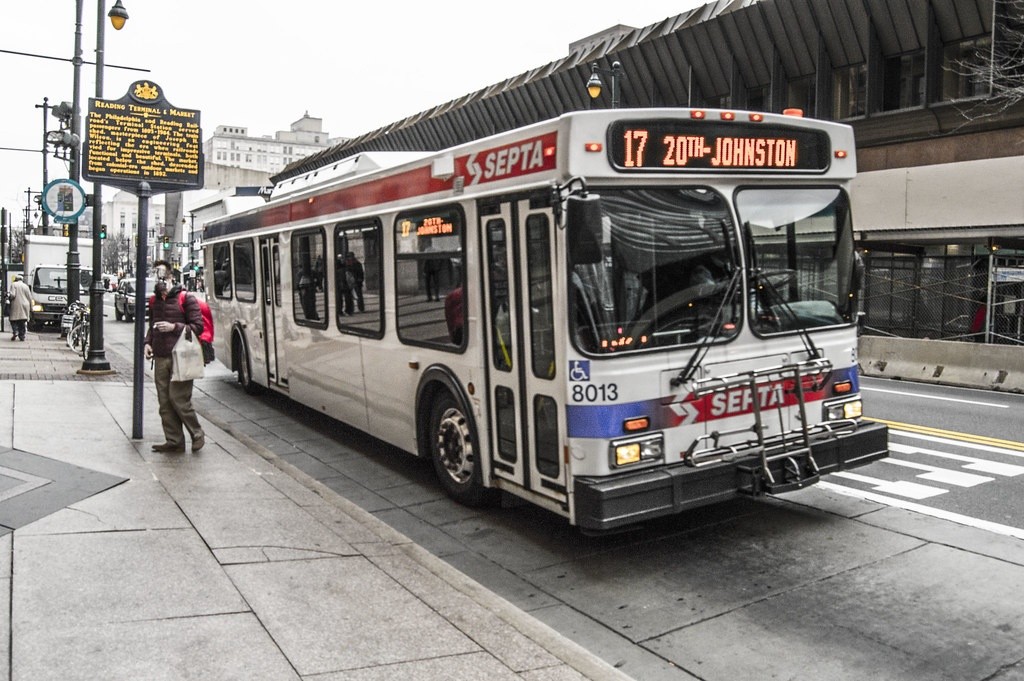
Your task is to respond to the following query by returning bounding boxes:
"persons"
[687,255,746,328]
[970,297,987,343]
[9,274,35,342]
[335,250,366,315]
[214,259,230,271]
[421,256,442,302]
[295,260,320,321]
[145,260,214,456]
[443,261,466,346]
[103,277,111,292]
[183,263,205,292]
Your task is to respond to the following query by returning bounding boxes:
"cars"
[101,271,118,292]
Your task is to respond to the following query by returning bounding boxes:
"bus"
[197,106,891,537]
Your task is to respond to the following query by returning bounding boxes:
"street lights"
[119,237,130,273]
[586,61,625,110]
[79,0,130,371]
[180,213,198,292]
[22,206,39,233]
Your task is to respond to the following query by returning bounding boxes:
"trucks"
[19,233,112,333]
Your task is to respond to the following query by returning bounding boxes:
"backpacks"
[149,289,215,366]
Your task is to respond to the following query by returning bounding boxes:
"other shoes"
[11,334,18,340]
[192,430,206,452]
[20,338,25,341]
[151,443,185,452]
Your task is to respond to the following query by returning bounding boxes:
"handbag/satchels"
[170,325,206,383]
[344,269,356,289]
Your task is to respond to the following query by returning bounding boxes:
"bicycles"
[58,300,110,361]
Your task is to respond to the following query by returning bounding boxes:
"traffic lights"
[162,236,170,248]
[100,224,108,240]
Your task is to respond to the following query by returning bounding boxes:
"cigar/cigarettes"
[153,324,158,329]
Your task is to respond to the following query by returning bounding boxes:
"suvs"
[113,277,167,323]
